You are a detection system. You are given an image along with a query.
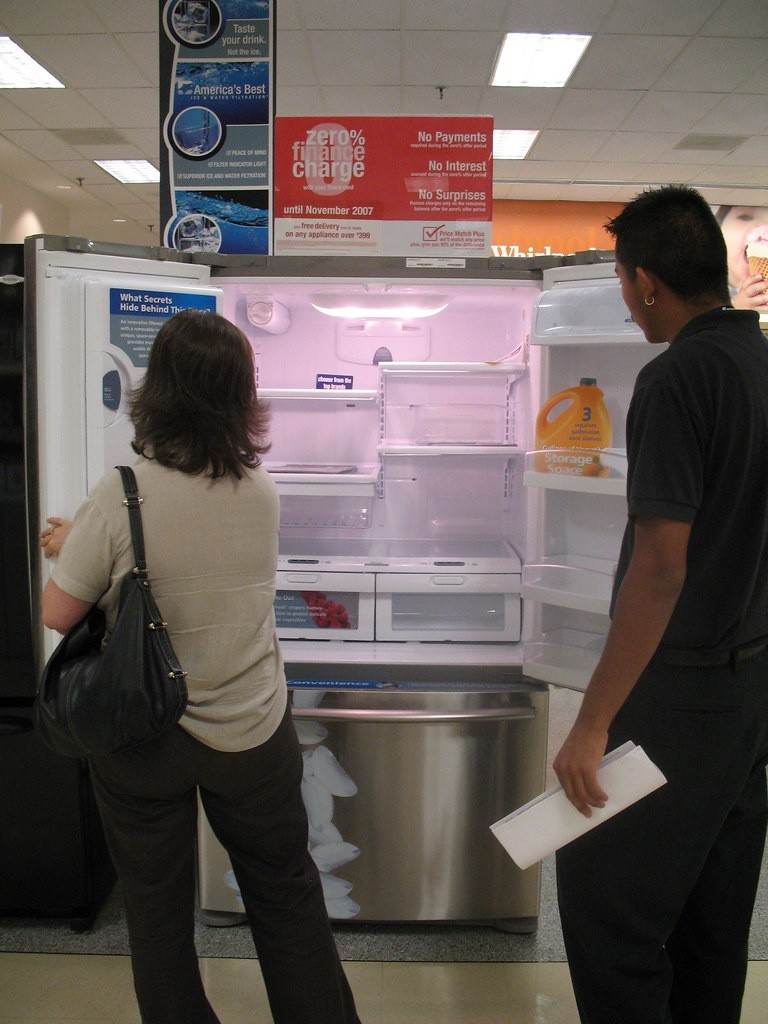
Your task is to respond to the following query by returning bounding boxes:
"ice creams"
[745,224,768,305]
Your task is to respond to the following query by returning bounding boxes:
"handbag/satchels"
[36,464,189,758]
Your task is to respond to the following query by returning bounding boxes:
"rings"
[50,524,59,534]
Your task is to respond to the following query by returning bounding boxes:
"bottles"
[534,377,613,480]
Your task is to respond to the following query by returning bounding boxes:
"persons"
[551,183,768,1024]
[36,308,361,1024]
[714,205,768,311]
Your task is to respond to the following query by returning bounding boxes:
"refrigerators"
[1,233,671,934]
